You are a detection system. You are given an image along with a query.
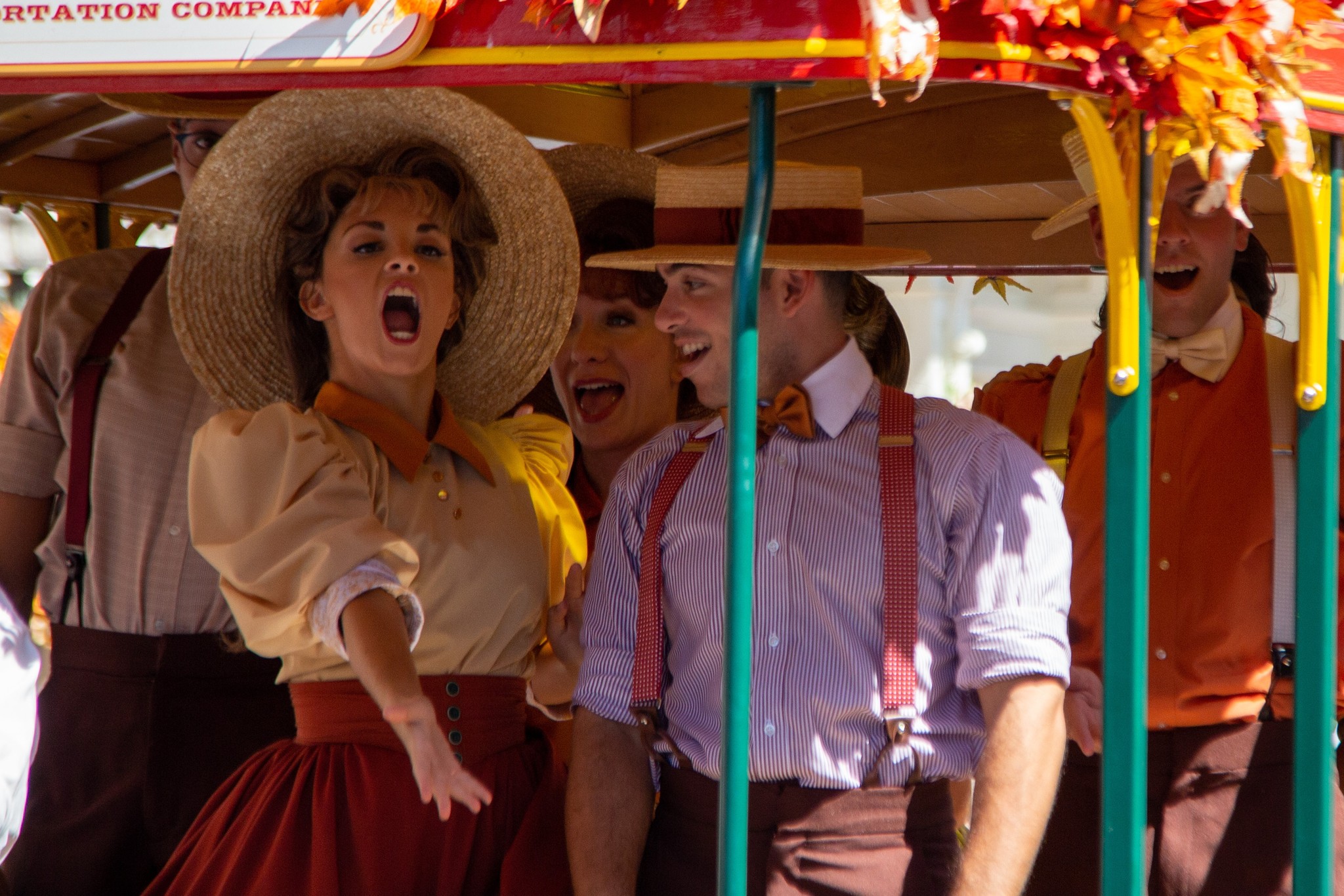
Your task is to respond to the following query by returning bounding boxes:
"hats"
[586,162,932,270]
[537,143,673,227]
[94,90,270,121]
[165,86,581,420]
[1031,121,1212,241]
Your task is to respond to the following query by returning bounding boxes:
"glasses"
[175,130,223,170]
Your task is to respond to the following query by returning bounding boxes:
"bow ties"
[721,383,815,449]
[1149,329,1229,383]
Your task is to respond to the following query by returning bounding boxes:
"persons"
[0,86,297,896]
[540,142,685,570]
[969,127,1343,896]
[141,87,592,896]
[566,164,1073,896]
